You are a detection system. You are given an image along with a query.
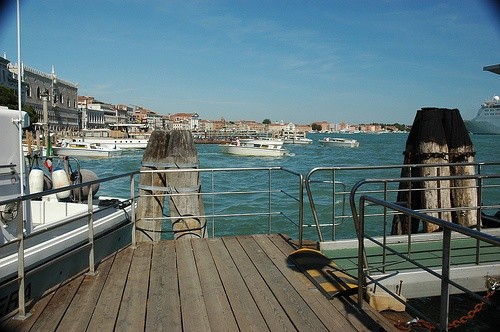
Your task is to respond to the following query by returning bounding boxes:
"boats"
[219,129,314,157]
[22,139,150,157]
[318,137,360,148]
[0,106,139,317]
[463,95,500,135]
